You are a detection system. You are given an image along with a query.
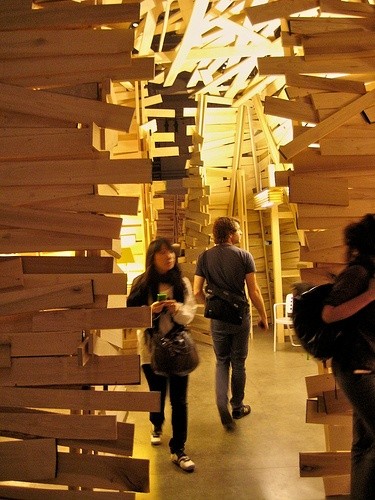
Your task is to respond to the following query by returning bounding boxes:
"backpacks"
[293,257,375,368]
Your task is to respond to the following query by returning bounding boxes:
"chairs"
[274,294,302,351]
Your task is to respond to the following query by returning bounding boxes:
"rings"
[170,303,174,307]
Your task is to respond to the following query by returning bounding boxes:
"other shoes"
[233,405,251,419]
[151,429,162,444]
[220,407,232,424]
[170,450,195,471]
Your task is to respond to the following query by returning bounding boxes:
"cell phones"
[157,293,167,301]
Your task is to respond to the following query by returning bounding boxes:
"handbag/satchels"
[203,282,247,326]
[144,307,200,377]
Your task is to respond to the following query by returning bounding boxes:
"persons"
[193,216,269,433]
[322,212,375,500]
[127,237,197,474]
